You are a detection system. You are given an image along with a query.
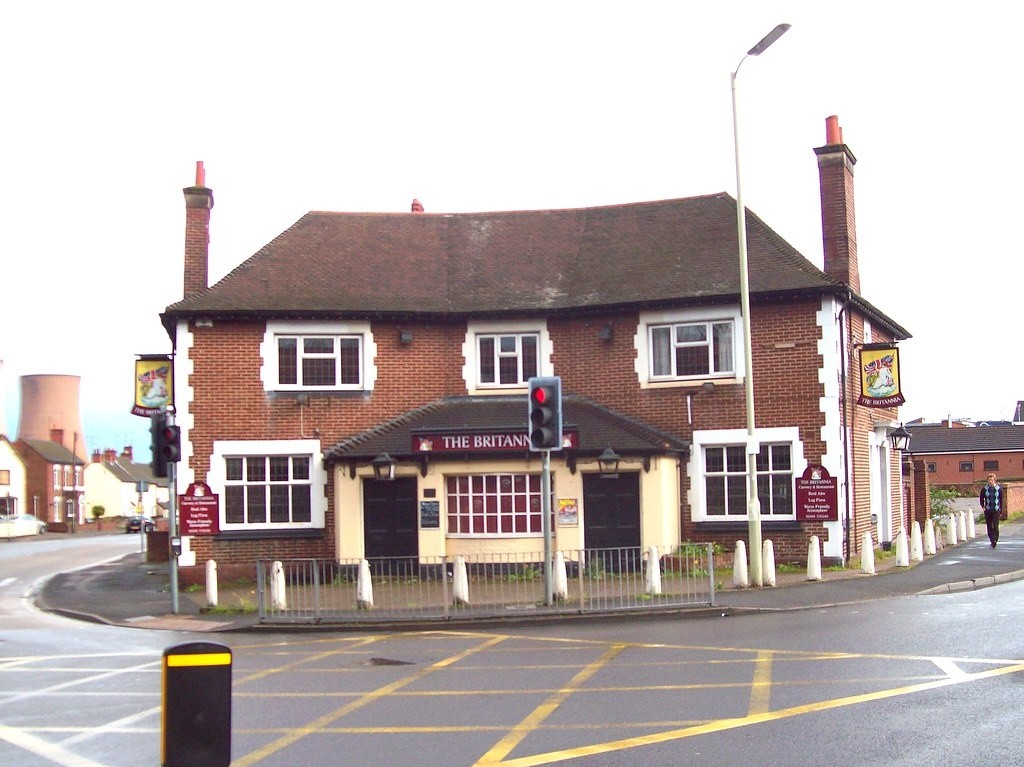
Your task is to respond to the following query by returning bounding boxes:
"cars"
[125,516,151,532]
[1,514,48,536]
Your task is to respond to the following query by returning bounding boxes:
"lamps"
[350,446,427,482]
[598,324,612,340]
[890,421,912,451]
[400,333,412,345]
[703,382,715,394]
[566,441,649,480]
[296,394,310,407]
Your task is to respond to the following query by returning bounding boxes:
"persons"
[980,473,1002,547]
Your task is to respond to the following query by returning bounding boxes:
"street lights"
[729,17,792,585]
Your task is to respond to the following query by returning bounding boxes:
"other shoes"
[992,540,996,547]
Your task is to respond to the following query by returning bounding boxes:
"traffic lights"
[527,378,563,452]
[162,425,182,463]
